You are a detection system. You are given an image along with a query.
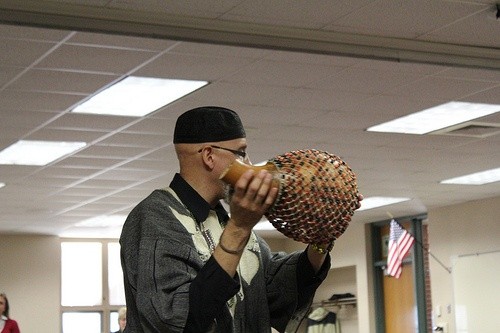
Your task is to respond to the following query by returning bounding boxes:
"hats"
[173,106,246,144]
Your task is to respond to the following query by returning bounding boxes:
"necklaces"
[204,230,235,308]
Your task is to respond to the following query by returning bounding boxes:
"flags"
[386,219,415,280]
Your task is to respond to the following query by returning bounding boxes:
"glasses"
[198,145,249,163]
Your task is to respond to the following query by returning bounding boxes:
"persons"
[114,308,128,333]
[0,292,20,333]
[119,106,334,333]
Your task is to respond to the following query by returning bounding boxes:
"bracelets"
[311,241,335,254]
[219,233,243,254]
